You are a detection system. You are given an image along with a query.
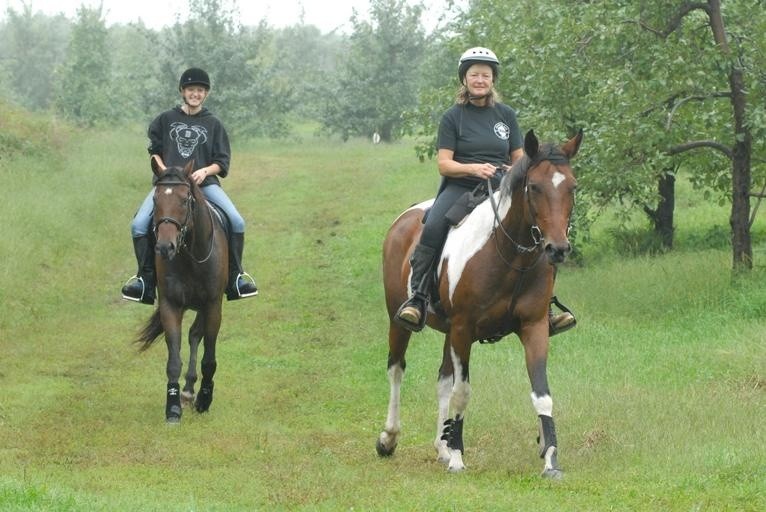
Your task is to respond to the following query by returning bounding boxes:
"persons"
[399,47,575,328]
[121,68,257,305]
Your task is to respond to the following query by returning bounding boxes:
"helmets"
[458,47,499,84]
[179,68,211,88]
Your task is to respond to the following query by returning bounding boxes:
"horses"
[374,125,584,481]
[130,156,233,428]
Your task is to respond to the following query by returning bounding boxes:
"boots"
[399,245,436,326]
[547,308,576,328]
[122,235,156,305]
[227,232,257,302]
